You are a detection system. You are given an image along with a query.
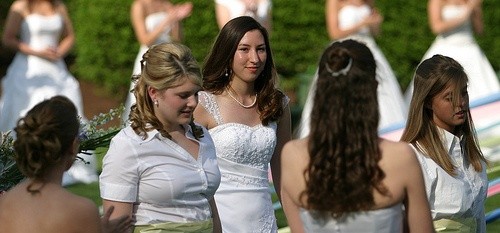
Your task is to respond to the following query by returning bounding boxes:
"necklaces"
[224,85,256,108]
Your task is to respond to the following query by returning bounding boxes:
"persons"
[122,0,193,124]
[404,0,500,132]
[193,16,293,233]
[279,39,435,233]
[99,42,221,233]
[297,0,404,141]
[0,96,136,233]
[399,54,488,233]
[215,0,273,33]
[0,0,98,188]
